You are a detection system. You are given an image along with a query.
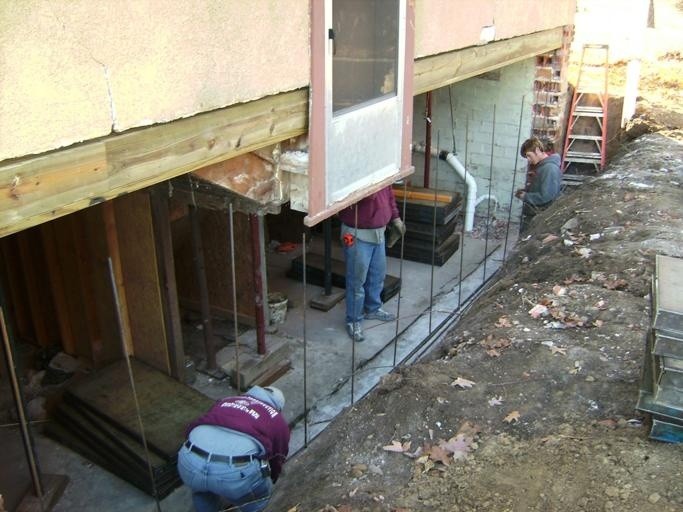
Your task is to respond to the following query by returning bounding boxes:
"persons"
[514,138,562,241]
[338,184,406,342]
[176,385,290,512]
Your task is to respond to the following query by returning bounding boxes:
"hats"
[262,386,285,412]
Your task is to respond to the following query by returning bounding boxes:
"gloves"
[391,218,406,235]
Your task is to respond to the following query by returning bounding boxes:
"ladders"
[560,43,609,194]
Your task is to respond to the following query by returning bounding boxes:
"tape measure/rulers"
[343,234,353,247]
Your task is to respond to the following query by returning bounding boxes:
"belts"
[184,439,257,463]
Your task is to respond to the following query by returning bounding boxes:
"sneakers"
[364,308,395,320]
[345,322,364,341]
